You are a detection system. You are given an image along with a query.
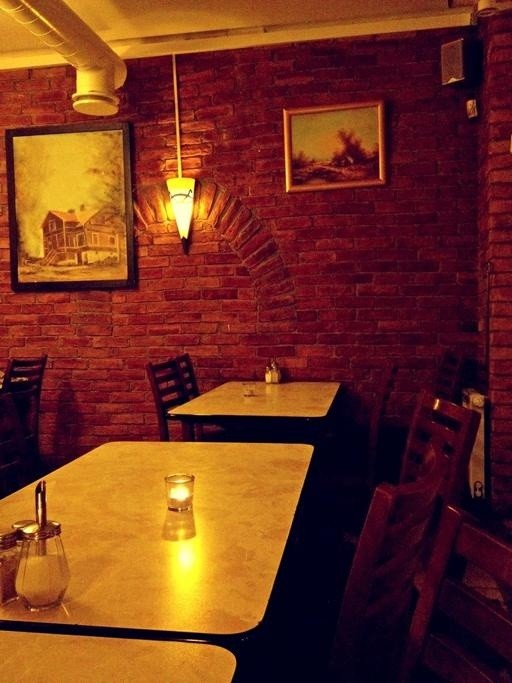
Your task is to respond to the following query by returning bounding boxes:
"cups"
[164,473,195,512]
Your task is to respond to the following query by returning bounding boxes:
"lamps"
[165,54,196,252]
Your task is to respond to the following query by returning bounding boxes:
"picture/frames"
[282,98,388,194]
[4,122,137,291]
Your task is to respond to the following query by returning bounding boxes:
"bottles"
[0,520,70,613]
[266,362,280,384]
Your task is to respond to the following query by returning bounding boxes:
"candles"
[245,389,253,395]
[169,485,191,508]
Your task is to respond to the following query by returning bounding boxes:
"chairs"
[344,366,399,455]
[434,351,467,402]
[0,354,49,487]
[396,504,512,683]
[398,392,479,487]
[169,354,204,439]
[147,358,191,442]
[334,447,446,650]
